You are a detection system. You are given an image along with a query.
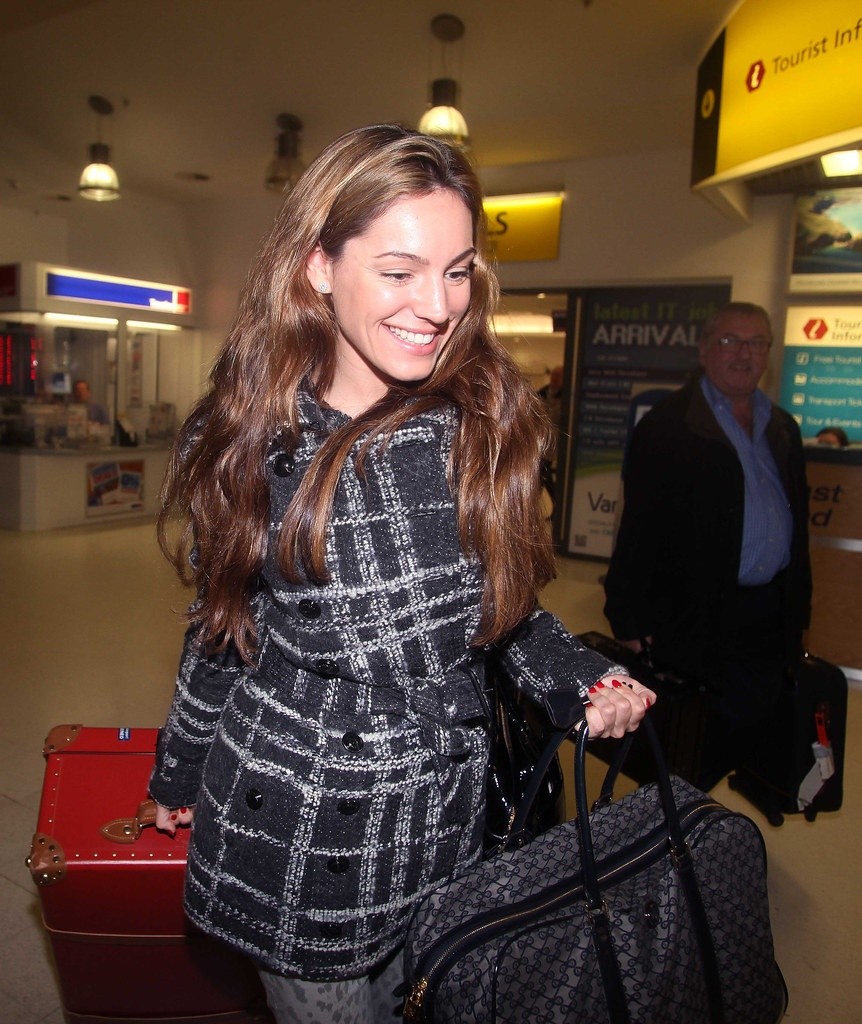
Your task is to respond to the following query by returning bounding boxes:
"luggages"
[728,648,848,827]
[24,723,275,1024]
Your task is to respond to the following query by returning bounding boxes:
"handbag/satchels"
[397,714,789,1024]
[478,658,566,862]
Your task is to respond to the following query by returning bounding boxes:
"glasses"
[708,335,769,362]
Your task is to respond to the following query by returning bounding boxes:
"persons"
[534,365,566,556]
[816,426,850,447]
[66,380,109,425]
[603,300,813,795]
[153,130,658,1024]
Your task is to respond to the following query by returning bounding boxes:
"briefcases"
[564,629,704,788]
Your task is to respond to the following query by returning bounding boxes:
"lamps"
[420,13,475,155]
[80,92,118,200]
